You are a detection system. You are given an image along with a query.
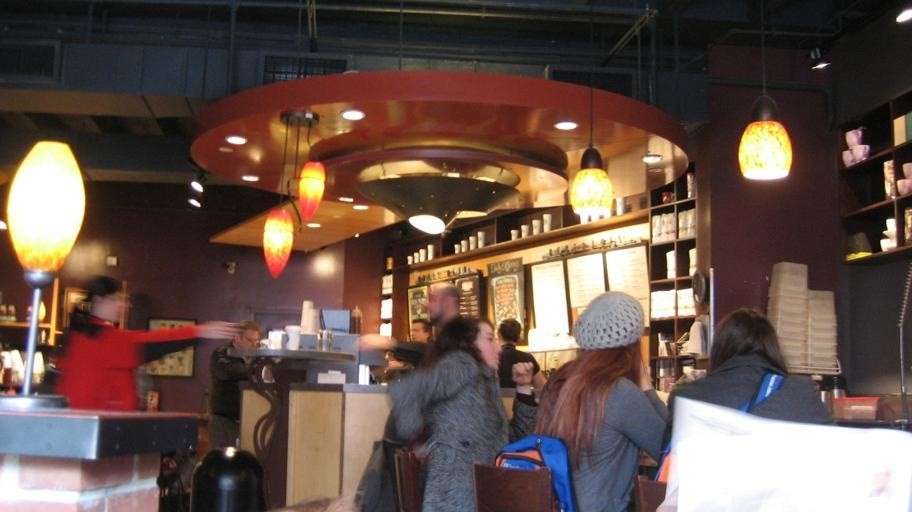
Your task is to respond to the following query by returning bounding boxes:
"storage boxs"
[766,259,840,373]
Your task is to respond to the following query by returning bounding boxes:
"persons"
[56,275,247,410]
[352,283,547,511]
[656,308,839,483]
[209,320,270,447]
[536,292,670,512]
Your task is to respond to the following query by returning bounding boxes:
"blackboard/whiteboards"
[488,256,525,342]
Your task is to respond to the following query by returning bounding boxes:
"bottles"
[350,305,362,334]
[814,375,846,402]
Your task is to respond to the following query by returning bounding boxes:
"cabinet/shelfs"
[406,191,650,267]
[647,182,699,387]
[837,88,911,263]
[378,241,407,341]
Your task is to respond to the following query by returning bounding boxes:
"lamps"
[568,3,615,217]
[0,139,86,410]
[263,101,331,277]
[734,3,796,184]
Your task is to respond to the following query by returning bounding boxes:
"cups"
[511,212,553,240]
[580,197,624,225]
[269,325,302,351]
[455,231,484,253]
[685,170,695,199]
[407,243,434,265]
[299,298,322,350]
[4,367,13,384]
[320,329,335,354]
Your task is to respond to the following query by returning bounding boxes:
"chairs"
[373,441,425,512]
[634,474,670,512]
[826,391,886,431]
[469,464,557,511]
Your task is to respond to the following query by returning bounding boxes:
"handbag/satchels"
[495,437,576,512]
[357,441,399,511]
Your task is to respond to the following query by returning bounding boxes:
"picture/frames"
[143,316,196,379]
[61,285,127,336]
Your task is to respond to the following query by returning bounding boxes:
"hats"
[574,292,645,349]
